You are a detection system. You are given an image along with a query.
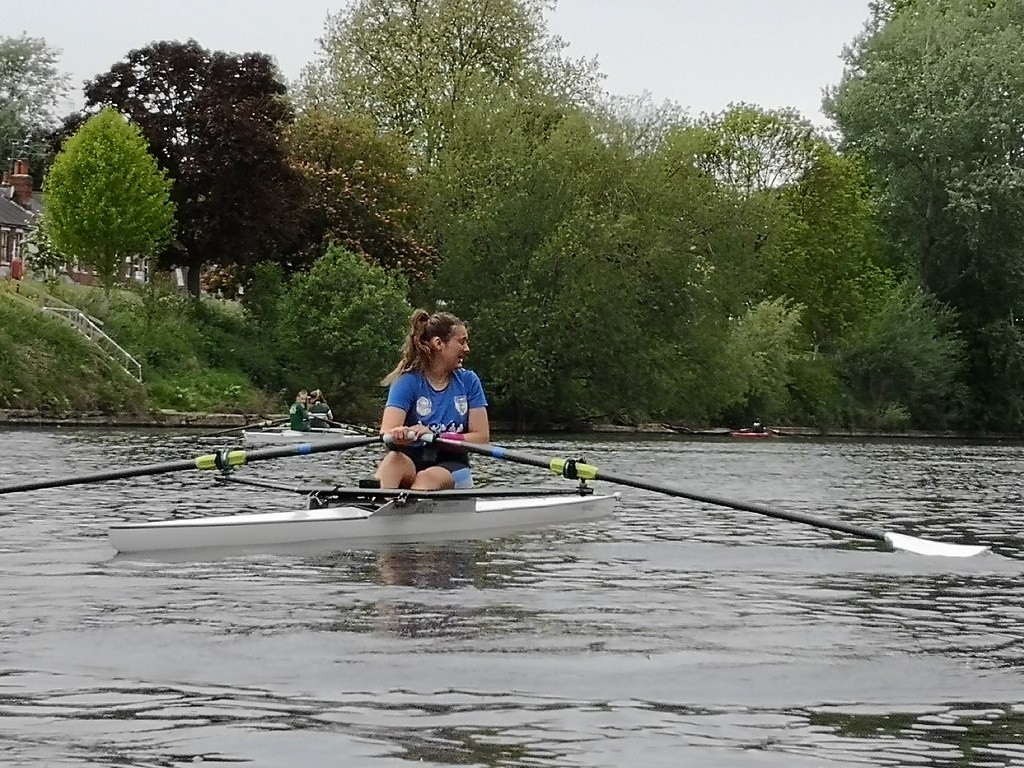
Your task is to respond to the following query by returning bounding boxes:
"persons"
[308,389,333,433]
[374,309,489,489]
[752,418,763,433]
[289,390,311,432]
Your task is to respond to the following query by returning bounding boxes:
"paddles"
[202,418,290,436]
[420,435,995,558]
[309,414,377,437]
[0,431,416,493]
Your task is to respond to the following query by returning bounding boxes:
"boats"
[730,431,773,439]
[241,429,367,444]
[107,493,623,554]
